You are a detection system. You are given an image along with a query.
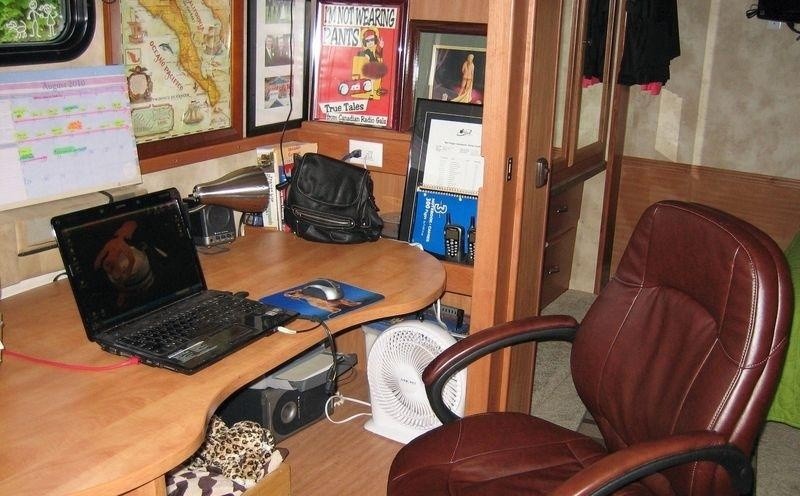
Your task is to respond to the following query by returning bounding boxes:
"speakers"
[214,384,335,446]
[184,199,236,246]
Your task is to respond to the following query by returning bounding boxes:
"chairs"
[386,201,794,496]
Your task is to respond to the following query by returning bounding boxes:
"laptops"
[49,186,301,376]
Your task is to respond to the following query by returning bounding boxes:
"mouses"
[303,277,344,301]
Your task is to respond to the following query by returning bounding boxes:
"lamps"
[182,166,270,214]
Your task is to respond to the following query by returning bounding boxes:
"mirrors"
[567,0,617,168]
[551,0,580,176]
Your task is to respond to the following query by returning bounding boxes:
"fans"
[363,319,468,445]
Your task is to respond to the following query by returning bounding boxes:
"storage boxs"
[538,180,584,312]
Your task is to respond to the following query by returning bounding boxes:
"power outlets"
[349,139,383,168]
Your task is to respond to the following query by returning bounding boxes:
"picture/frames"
[308,0,409,131]
[103,0,244,160]
[0,0,96,67]
[247,0,311,138]
[400,19,488,133]
[397,97,483,266]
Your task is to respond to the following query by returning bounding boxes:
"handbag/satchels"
[277,152,384,244]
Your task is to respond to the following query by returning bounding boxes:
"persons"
[265,35,290,66]
[451,53,476,104]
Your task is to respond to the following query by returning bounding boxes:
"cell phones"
[468,216,476,265]
[442,213,464,264]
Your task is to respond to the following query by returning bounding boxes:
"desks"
[0,223,447,496]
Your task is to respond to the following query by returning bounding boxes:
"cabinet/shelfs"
[549,0,619,197]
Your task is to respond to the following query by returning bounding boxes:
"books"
[256,141,318,231]
